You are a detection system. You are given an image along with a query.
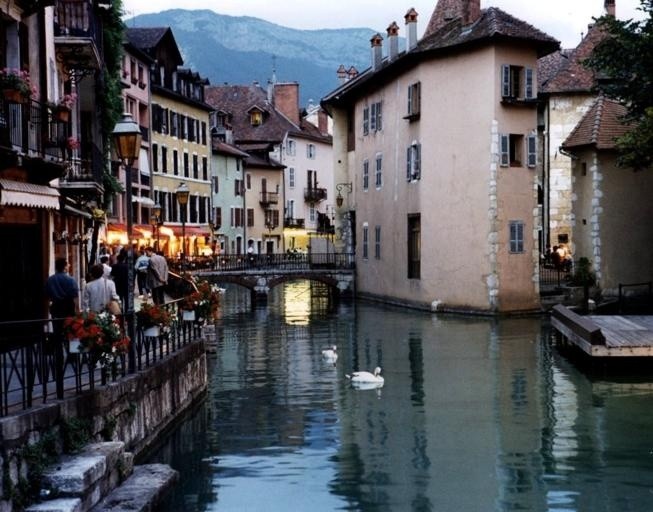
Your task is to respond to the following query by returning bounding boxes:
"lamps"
[334,179,353,209]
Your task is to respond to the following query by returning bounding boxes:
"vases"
[182,307,198,322]
[54,105,71,123]
[66,337,90,356]
[142,322,164,338]
[3,87,30,105]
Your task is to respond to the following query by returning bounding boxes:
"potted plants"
[564,257,603,303]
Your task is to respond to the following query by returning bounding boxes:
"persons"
[287,247,301,259]
[247,246,253,260]
[45,244,170,368]
[546,244,566,266]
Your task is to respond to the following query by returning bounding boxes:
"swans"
[320,345,337,356]
[344,366,385,382]
[346,381,385,400]
[322,356,338,368]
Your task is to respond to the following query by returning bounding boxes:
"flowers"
[58,90,79,109]
[184,281,226,314]
[137,303,179,325]
[61,309,130,366]
[0,67,37,93]
[45,132,83,152]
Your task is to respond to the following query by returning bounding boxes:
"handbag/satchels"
[107,300,120,314]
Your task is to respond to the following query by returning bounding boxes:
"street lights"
[150,202,162,254]
[208,221,215,241]
[267,220,273,244]
[109,111,144,375]
[174,181,190,259]
[149,214,156,240]
